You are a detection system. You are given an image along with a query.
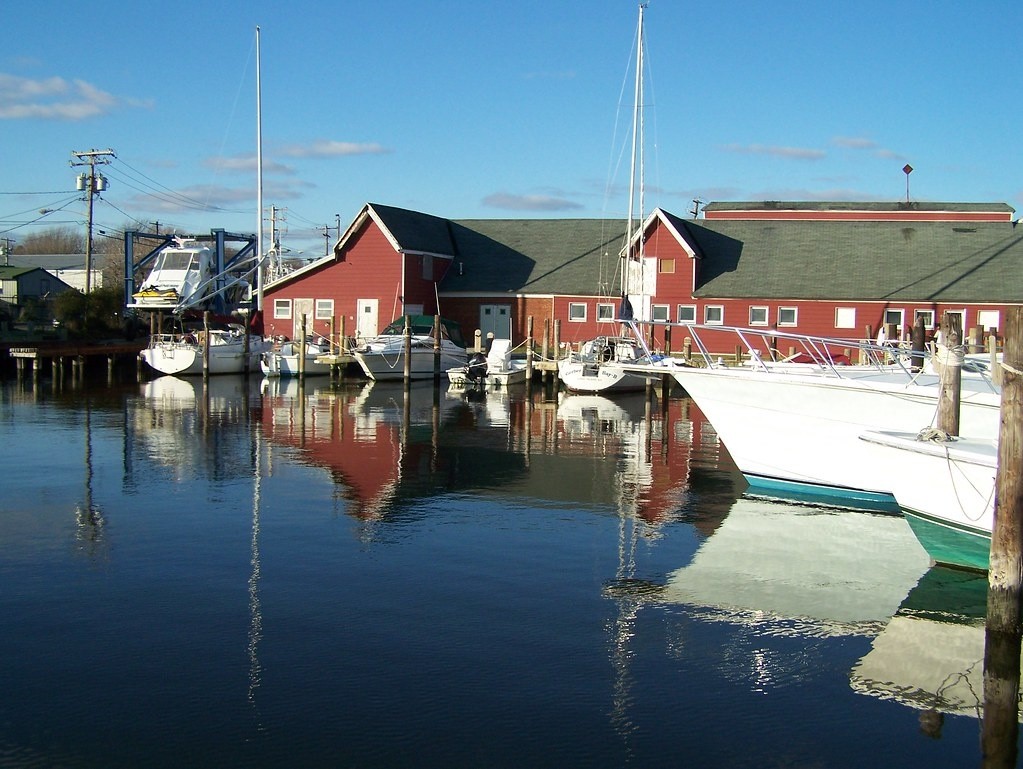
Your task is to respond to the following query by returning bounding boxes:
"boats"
[131,235,248,309]
[614,318,1013,513]
[140,326,272,374]
[606,500,934,637]
[852,566,1023,729]
[859,430,996,574]
[260,333,353,377]
[445,339,536,386]
[350,279,464,382]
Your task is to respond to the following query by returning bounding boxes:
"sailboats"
[558,381,659,727]
[557,4,661,393]
